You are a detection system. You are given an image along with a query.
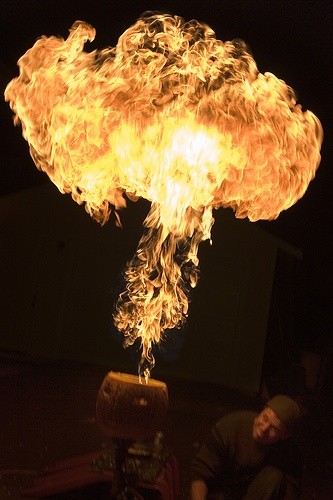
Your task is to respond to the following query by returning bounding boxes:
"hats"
[268,395,300,433]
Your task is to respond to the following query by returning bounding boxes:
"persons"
[188,394,302,500]
